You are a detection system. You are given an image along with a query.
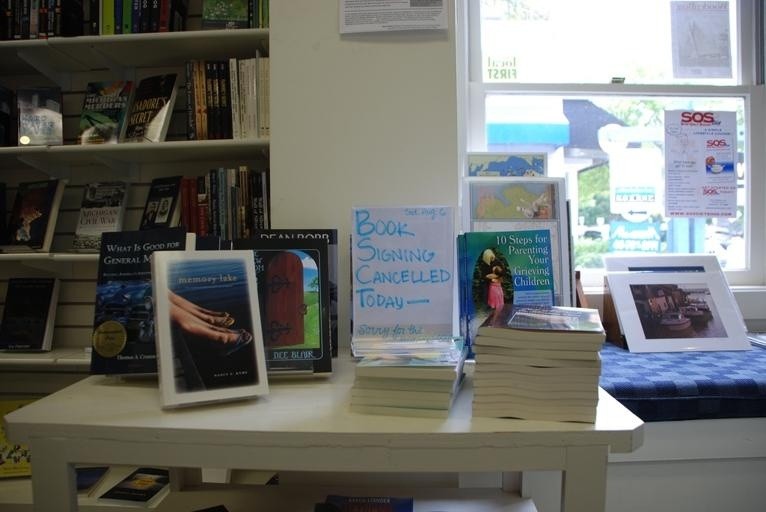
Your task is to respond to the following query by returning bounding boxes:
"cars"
[96,285,121,309]
[100,283,154,343]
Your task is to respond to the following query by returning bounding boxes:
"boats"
[690,296,710,311]
[678,305,704,316]
[659,312,691,335]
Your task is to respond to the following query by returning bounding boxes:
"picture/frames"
[604,266,755,353]
[604,253,749,331]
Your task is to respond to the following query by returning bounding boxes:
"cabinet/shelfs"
[1,19,270,376]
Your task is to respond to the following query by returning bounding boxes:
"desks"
[3,358,645,510]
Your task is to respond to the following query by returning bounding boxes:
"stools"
[593,337,766,510]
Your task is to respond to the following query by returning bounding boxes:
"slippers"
[216,311,252,358]
